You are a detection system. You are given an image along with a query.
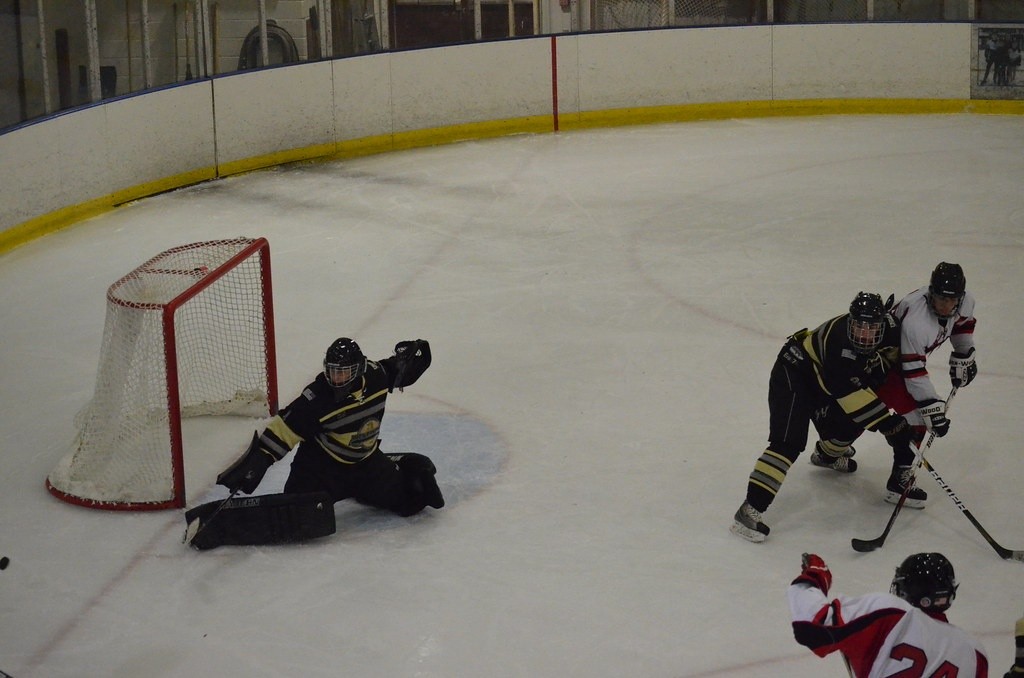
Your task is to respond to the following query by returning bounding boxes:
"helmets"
[323,337,365,392]
[889,552,955,612]
[929,262,966,298]
[847,291,885,356]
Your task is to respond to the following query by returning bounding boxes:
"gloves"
[878,413,916,451]
[791,553,832,597]
[917,399,951,437]
[949,347,978,387]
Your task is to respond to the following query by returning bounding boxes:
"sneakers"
[811,441,858,472]
[883,464,927,509]
[729,500,770,543]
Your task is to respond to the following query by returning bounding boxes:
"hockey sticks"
[802,552,854,676]
[180,490,235,551]
[909,443,1024,562]
[851,386,957,553]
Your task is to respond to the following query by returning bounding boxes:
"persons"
[729,292,928,546]
[182,336,445,554]
[785,553,1024,677]
[882,261,980,463]
[980,32,1022,85]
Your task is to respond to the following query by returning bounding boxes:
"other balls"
[0,556,9,571]
